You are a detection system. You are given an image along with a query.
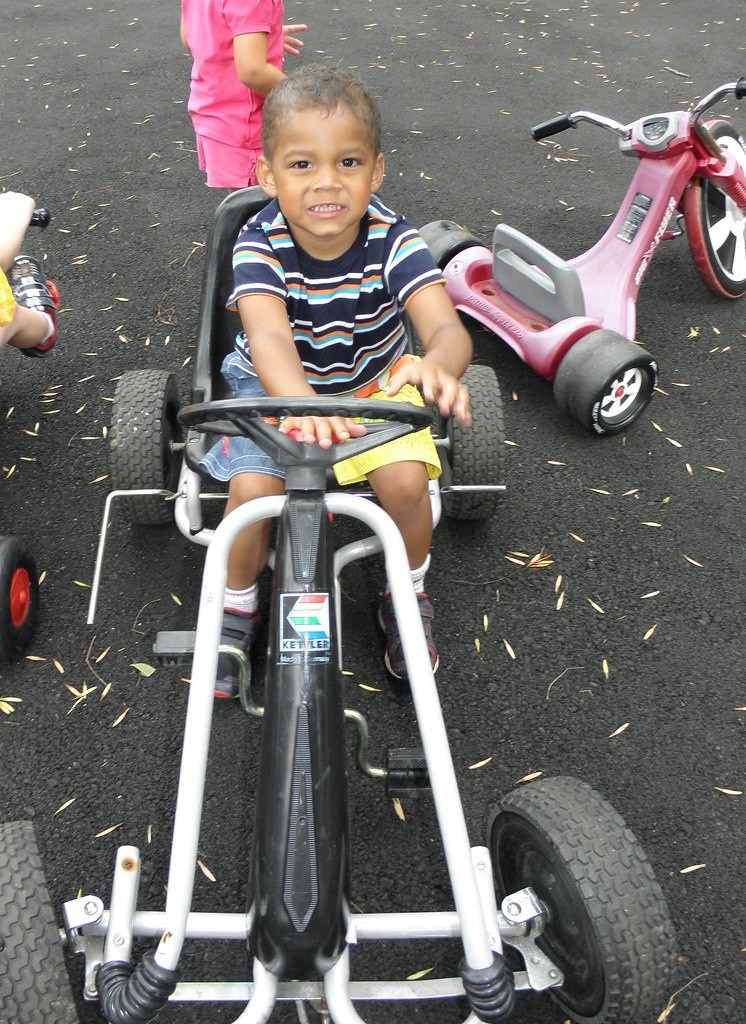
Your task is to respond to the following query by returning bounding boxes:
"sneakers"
[215,608,261,700]
[4,254,59,359]
[377,593,440,680]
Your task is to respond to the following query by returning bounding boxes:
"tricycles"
[415,77,745,438]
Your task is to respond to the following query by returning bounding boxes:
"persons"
[0,191,60,359]
[197,64,472,700]
[180,0,308,196]
